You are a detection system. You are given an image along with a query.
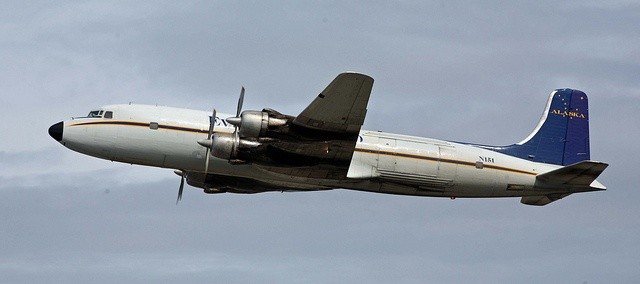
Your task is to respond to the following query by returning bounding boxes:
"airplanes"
[48,72,609,206]
